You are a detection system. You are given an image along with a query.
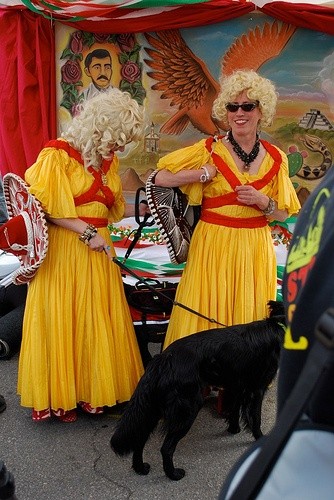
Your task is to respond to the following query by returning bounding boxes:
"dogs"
[106,300,288,481]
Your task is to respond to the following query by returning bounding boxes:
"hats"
[145,168,201,264]
[0,173,48,287]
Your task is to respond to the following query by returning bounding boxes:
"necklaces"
[97,165,107,184]
[228,130,260,171]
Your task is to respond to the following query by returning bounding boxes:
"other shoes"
[57,412,77,423]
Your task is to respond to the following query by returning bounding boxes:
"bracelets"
[261,198,275,216]
[200,166,210,183]
[78,224,97,246]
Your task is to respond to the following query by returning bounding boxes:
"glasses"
[225,101,259,112]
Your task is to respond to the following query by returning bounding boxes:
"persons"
[16,89,145,422]
[155,71,302,417]
